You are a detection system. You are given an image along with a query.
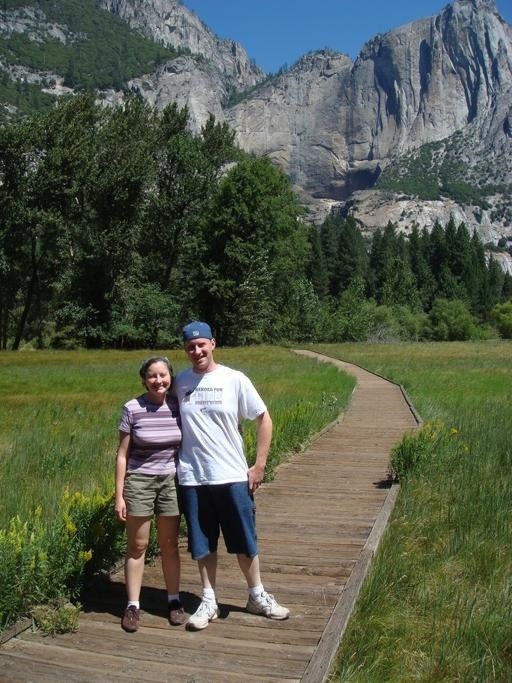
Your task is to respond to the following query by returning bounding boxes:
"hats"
[182,320,212,342]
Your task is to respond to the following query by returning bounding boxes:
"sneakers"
[185,601,221,630]
[122,605,141,632]
[245,591,290,620]
[168,598,187,625]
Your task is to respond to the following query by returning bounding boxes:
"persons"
[114,357,245,632]
[116,321,290,632]
